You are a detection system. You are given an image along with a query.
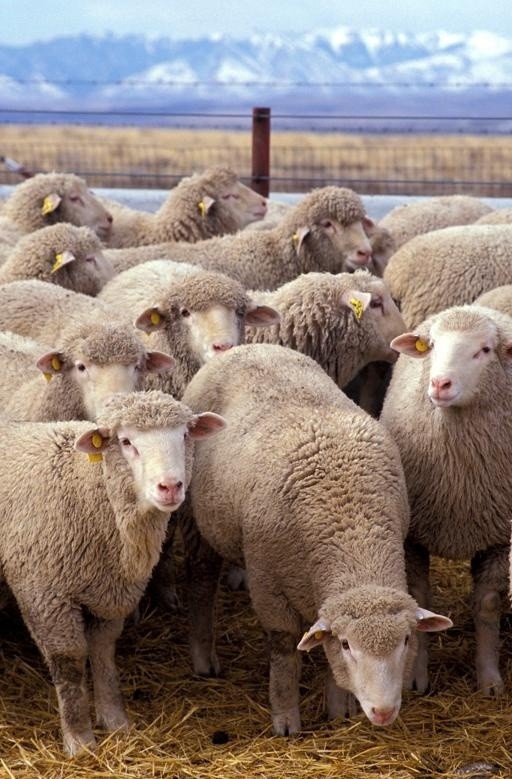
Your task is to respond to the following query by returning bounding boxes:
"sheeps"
[0,167,512,760]
